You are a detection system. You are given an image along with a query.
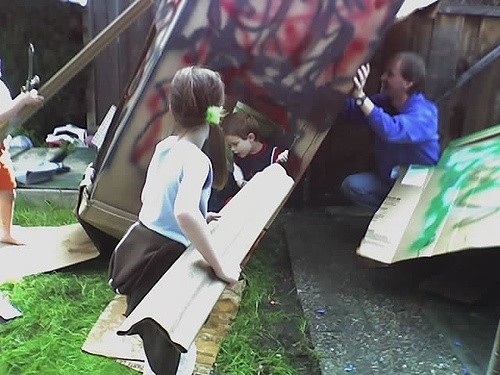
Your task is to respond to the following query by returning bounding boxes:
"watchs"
[354,94,368,108]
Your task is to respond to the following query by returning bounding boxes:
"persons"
[218,111,290,208]
[0,88,44,126]
[340,51,441,269]
[106,67,242,375]
[1,64,28,246]
